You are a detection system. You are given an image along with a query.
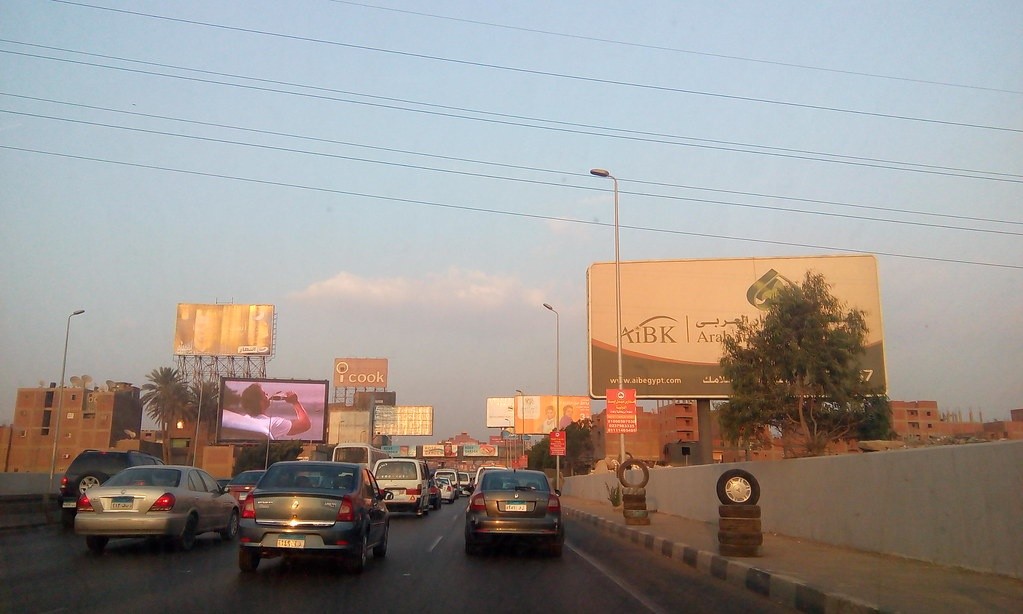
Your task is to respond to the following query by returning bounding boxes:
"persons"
[543,406,556,434]
[560,406,574,429]
[242,383,311,440]
[444,445,452,456]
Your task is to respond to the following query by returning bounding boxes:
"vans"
[373,457,432,517]
[435,468,460,498]
[475,467,505,484]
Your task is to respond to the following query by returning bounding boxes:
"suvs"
[58,449,165,517]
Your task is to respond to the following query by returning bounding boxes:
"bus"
[333,443,393,471]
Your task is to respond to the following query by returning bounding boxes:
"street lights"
[543,303,559,489]
[265,391,282,470]
[589,168,624,509]
[49,309,86,496]
[192,363,214,467]
[338,420,345,444]
[505,418,512,469]
[516,390,525,470]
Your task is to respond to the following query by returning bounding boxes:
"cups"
[269,389,288,405]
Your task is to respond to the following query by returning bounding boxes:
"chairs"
[526,483,540,491]
[490,478,503,488]
[295,476,312,488]
[335,475,353,489]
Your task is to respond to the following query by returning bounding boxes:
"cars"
[434,477,455,503]
[74,464,240,553]
[429,472,441,510]
[224,469,268,515]
[464,468,563,558]
[239,460,393,574]
[457,472,473,496]
